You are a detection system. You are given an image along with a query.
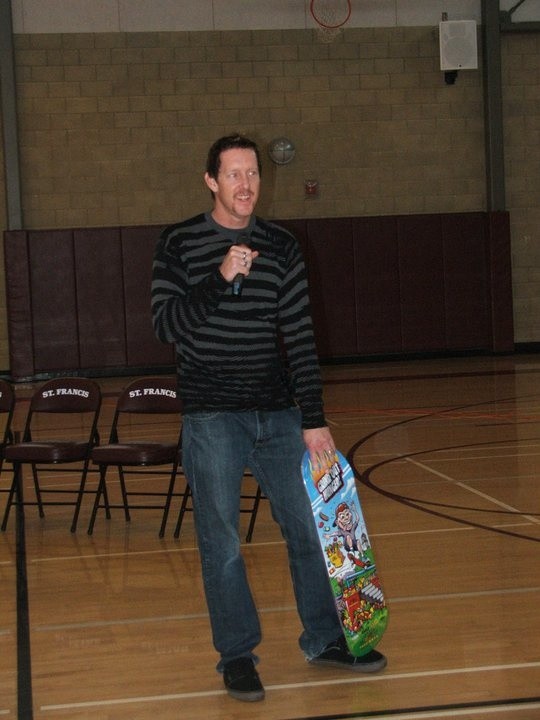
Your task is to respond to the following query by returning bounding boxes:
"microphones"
[233,232,252,297]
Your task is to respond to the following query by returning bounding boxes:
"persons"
[146,133,388,701]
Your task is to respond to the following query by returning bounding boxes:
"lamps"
[269,139,297,165]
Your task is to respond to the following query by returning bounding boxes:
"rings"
[243,251,247,260]
[244,260,248,267]
[326,449,335,456]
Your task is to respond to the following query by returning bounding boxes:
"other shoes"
[223,657,265,701]
[309,634,387,673]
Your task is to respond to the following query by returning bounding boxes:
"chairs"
[5,377,113,534]
[87,378,192,537]
[0,378,24,533]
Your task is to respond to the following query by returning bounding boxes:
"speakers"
[440,21,477,71]
